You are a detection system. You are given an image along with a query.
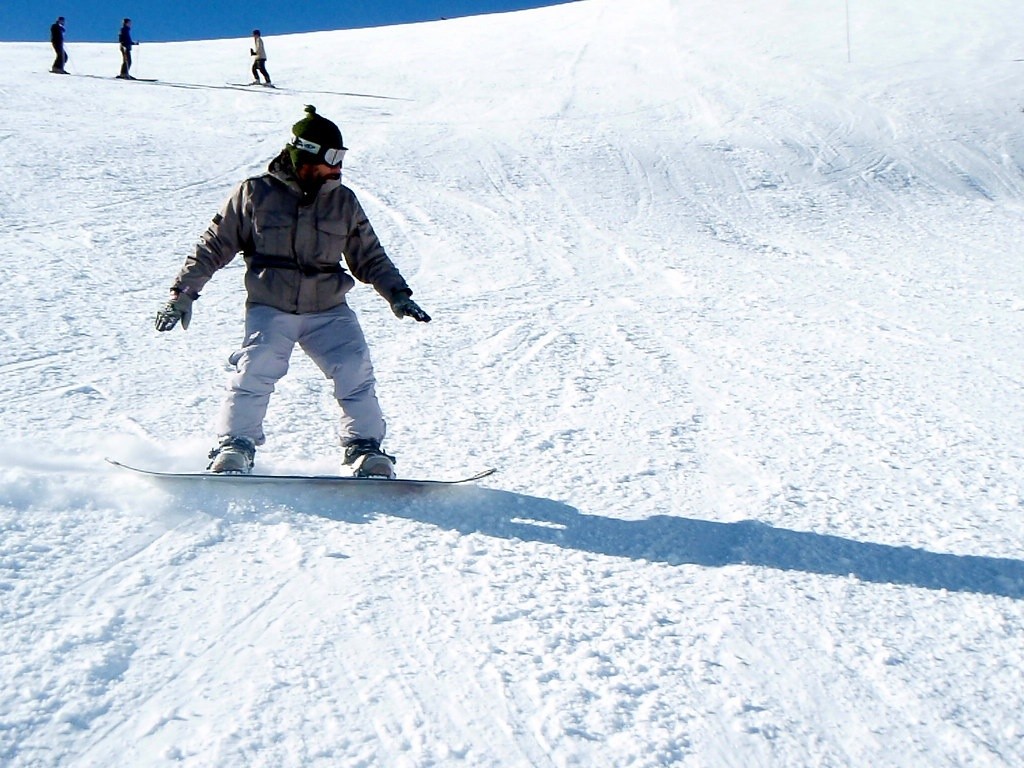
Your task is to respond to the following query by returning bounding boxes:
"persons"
[50,17,68,72]
[118,18,139,77]
[250,29,271,86]
[154,104,432,478]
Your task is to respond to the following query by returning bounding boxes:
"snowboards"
[106,458,498,484]
[48,70,72,75]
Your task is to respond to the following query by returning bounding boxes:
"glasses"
[325,147,346,166]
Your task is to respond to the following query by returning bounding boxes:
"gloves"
[389,291,431,323]
[154,291,194,332]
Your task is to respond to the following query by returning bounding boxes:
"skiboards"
[109,76,158,82]
[225,82,287,90]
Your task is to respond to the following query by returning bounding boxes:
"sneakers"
[341,438,397,479]
[206,435,257,473]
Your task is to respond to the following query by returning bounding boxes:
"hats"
[286,105,349,175]
